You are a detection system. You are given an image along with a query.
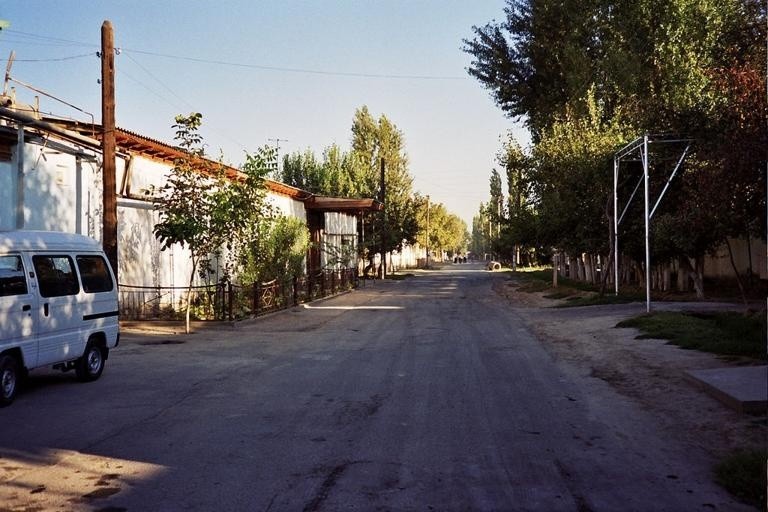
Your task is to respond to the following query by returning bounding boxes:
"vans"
[0,228,120,406]
